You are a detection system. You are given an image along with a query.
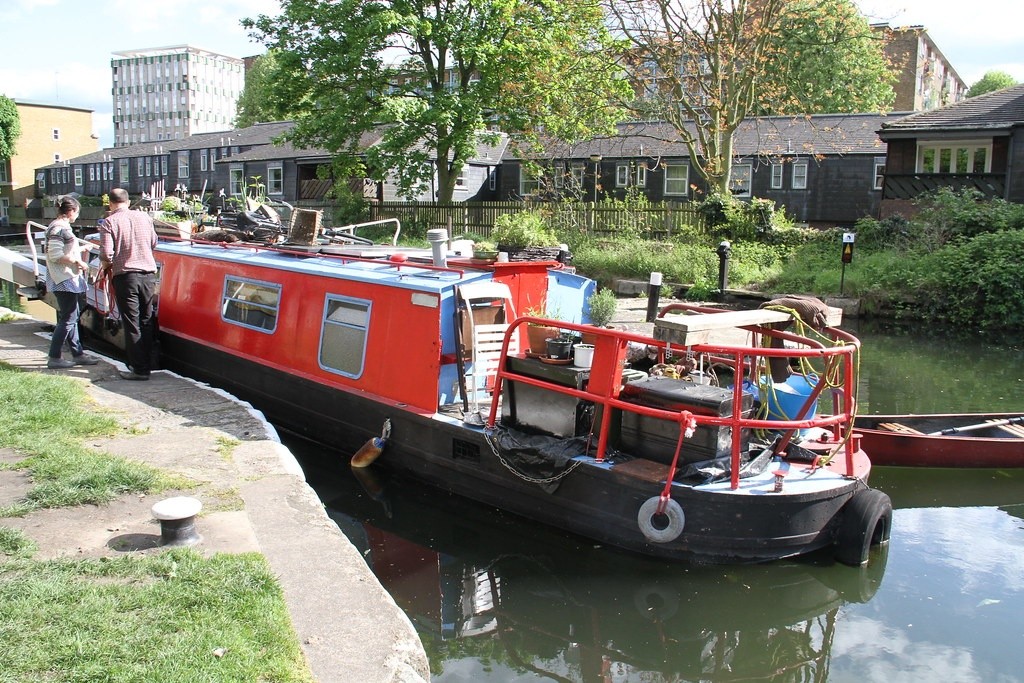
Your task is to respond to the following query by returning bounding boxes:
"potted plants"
[472,240,499,258]
[103,175,273,241]
[543,314,578,359]
[522,284,566,353]
[561,315,582,357]
[580,286,617,343]
[491,208,562,262]
[573,344,595,367]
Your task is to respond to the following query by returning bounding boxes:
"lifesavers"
[636,496,686,544]
[832,486,894,565]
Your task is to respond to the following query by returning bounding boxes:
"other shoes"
[72,354,98,365]
[119,370,149,381]
[128,365,135,371]
[48,358,76,367]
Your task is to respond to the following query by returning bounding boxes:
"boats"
[0,204,893,567]
[844,412,1024,467]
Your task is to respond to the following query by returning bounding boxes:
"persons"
[99,188,158,381]
[45,196,99,368]
